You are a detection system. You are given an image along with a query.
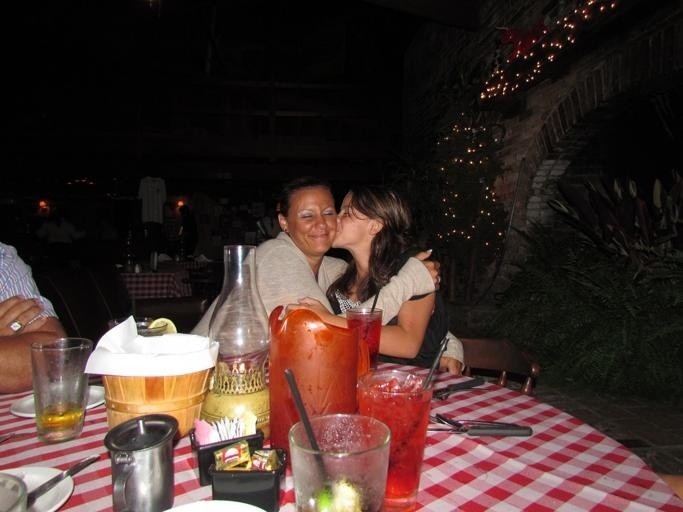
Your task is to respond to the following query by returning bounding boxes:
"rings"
[9,318,23,334]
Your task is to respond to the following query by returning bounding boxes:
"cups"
[0,473,28,512]
[29,338,93,442]
[103,414,179,512]
[289,413,392,512]
[108,317,168,337]
[346,307,383,370]
[358,369,435,512]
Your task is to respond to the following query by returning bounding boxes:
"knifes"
[427,424,533,437]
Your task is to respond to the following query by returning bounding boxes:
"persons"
[286,181,446,368]
[0,231,67,397]
[189,175,465,374]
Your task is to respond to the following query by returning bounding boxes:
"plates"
[0,467,74,512]
[9,385,105,418]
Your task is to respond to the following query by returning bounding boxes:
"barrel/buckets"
[96,333,219,440]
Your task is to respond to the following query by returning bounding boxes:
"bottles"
[199,245,271,433]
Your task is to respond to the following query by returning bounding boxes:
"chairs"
[449,336,539,399]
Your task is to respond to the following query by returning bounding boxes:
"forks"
[436,413,515,426]
